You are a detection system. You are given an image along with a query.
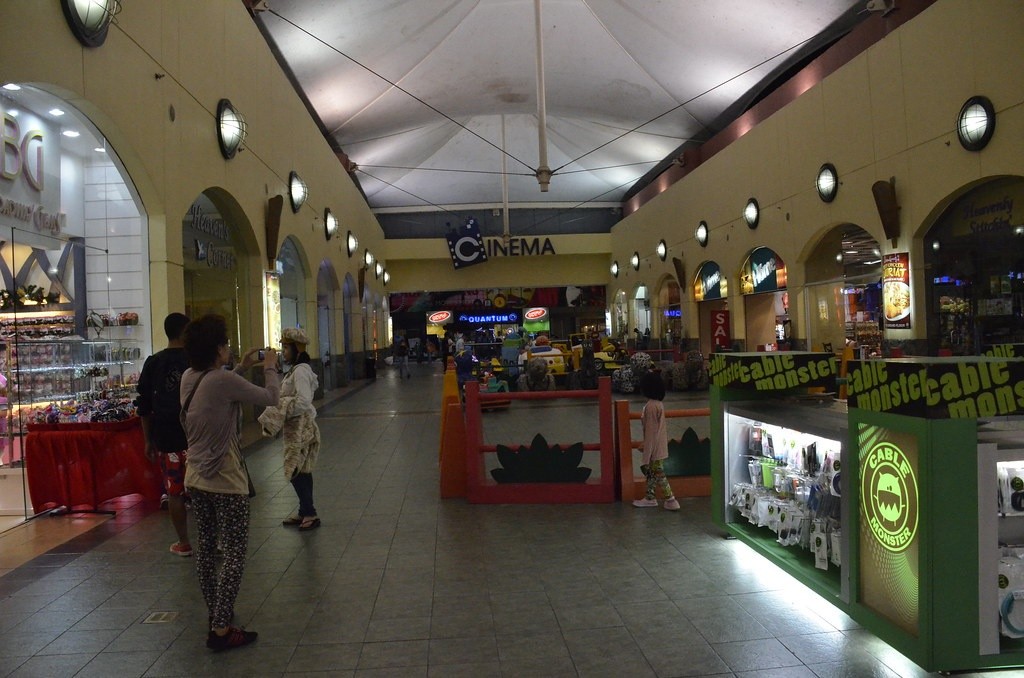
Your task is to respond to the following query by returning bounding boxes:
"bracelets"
[264,368,276,373]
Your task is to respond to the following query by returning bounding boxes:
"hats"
[278,327,311,346]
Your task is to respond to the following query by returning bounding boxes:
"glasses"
[223,337,231,348]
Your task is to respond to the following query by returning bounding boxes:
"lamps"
[347,230,359,258]
[365,249,390,285]
[956,95,996,151]
[743,198,759,230]
[289,171,310,212]
[657,240,666,259]
[610,261,619,278]
[631,252,639,271]
[216,98,249,159]
[694,220,708,247]
[816,163,838,202]
[324,207,340,240]
[61,0,123,49]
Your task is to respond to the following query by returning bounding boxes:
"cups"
[853,348,860,359]
[891,347,901,357]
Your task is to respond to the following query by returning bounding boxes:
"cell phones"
[258,349,265,360]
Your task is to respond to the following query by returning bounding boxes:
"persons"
[633,373,680,510]
[396,339,411,380]
[180,314,280,650]
[442,328,504,373]
[258,327,321,531]
[634,328,650,344]
[136,313,223,556]
[414,338,434,368]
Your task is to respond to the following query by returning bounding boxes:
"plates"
[525,308,546,319]
[429,311,450,322]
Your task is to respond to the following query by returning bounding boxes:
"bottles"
[874,347,881,354]
[861,345,869,359]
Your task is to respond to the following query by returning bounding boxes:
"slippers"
[298,517,320,530]
[283,514,303,524]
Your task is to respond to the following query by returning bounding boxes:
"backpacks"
[140,354,187,452]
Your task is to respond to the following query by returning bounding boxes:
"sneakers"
[632,498,658,507]
[169,542,193,556]
[663,495,680,511]
[159,495,170,510]
[207,622,258,649]
